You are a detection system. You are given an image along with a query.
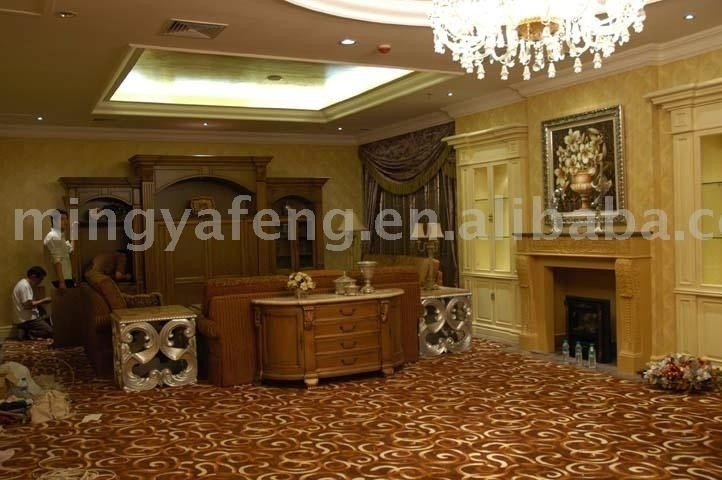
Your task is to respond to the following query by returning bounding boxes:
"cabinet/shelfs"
[252,294,403,389]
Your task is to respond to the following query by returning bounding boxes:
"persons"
[11,208,80,339]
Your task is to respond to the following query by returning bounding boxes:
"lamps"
[411,222,446,288]
[421,1,648,83]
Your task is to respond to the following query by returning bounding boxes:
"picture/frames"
[539,102,628,225]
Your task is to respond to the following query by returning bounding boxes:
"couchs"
[187,265,420,388]
[361,253,444,287]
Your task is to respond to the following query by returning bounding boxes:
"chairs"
[77,269,163,379]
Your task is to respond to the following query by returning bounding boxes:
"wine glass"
[359,261,376,295]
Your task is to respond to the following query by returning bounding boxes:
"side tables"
[420,285,472,358]
[108,305,200,395]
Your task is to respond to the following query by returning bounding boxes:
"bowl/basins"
[334,274,356,294]
[344,285,361,296]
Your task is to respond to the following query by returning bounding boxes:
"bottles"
[562,339,572,367]
[575,341,583,368]
[588,343,597,368]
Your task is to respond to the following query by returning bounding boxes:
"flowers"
[285,270,316,297]
[641,351,721,391]
